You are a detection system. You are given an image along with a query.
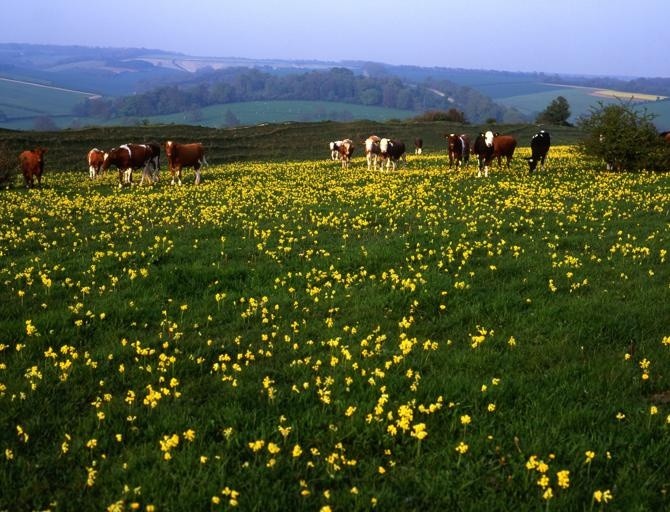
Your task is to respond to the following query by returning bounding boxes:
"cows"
[18,148,49,189]
[414,137,423,155]
[443,132,470,172]
[87,143,161,188]
[474,131,499,178]
[159,140,209,187]
[523,130,551,174]
[362,136,406,172]
[471,135,519,170]
[328,139,354,169]
